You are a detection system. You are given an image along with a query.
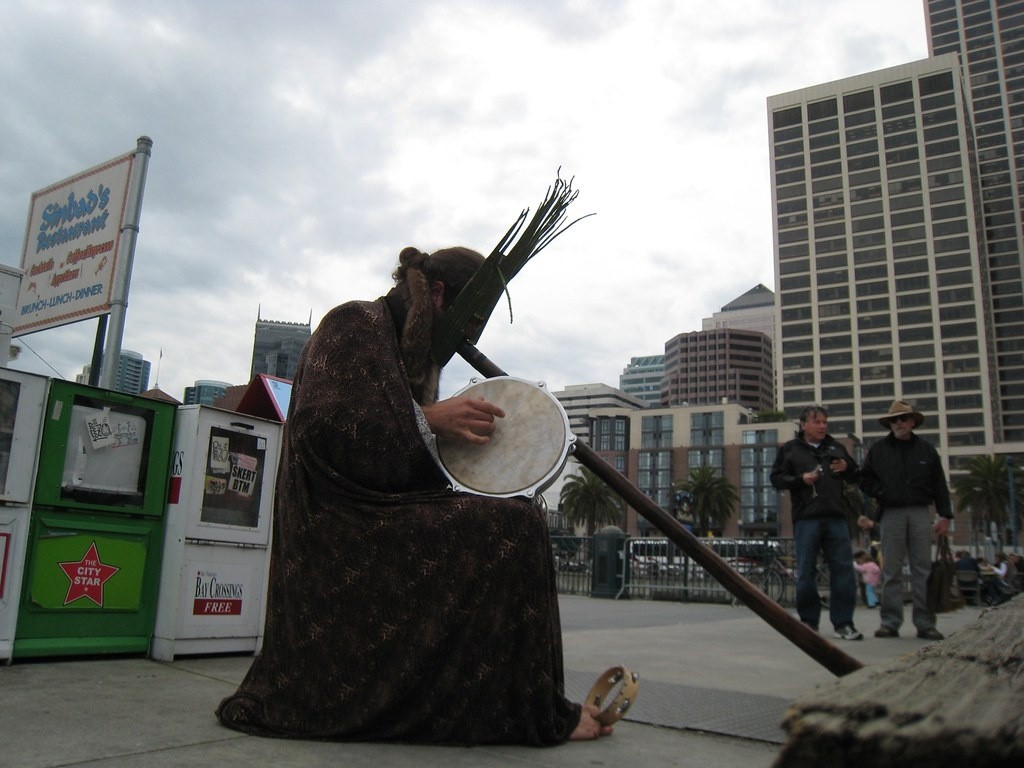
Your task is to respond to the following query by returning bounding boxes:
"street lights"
[1004,454,1019,553]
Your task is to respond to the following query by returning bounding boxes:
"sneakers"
[832,625,863,640]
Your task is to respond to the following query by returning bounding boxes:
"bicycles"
[746,544,831,608]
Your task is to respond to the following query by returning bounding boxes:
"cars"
[549,527,587,571]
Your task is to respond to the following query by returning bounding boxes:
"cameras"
[813,464,824,473]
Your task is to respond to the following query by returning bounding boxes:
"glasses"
[889,414,908,423]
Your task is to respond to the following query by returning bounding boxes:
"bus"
[628,538,778,578]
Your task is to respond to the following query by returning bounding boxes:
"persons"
[954,550,1024,607]
[214,246,614,750]
[853,540,882,608]
[859,401,955,640]
[769,405,864,639]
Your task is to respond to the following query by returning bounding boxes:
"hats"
[879,401,925,429]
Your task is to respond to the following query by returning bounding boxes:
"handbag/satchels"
[926,535,963,613]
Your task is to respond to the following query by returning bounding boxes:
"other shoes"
[875,626,898,637]
[917,627,944,640]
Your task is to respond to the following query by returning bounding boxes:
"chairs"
[956,570,981,605]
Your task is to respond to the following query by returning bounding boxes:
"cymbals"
[584,665,641,727]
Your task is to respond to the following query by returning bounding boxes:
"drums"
[436,379,566,493]
